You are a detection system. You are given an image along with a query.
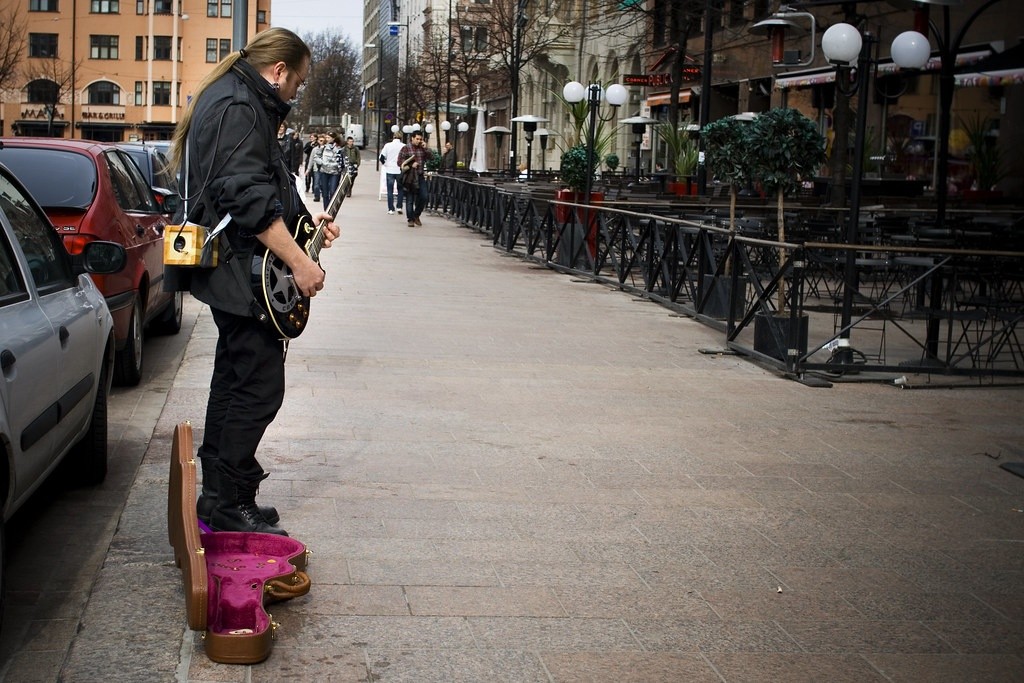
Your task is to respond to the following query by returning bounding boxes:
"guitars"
[251,158,359,342]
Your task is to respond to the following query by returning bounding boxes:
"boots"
[196,457,279,526]
[208,460,289,538]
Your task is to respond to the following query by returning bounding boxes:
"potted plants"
[700,116,755,322]
[651,119,699,198]
[555,146,604,263]
[739,108,826,362]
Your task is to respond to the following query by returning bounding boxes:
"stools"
[595,192,1024,383]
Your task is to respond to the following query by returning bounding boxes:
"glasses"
[286,64,306,91]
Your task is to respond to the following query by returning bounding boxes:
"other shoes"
[397,208,403,214]
[407,218,414,227]
[386,210,394,215]
[413,216,422,226]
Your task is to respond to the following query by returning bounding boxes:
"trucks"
[345,124,363,148]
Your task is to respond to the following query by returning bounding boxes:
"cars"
[0,135,185,388]
[0,159,128,539]
[104,137,182,217]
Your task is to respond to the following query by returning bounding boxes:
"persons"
[438,143,457,200]
[154,28,339,536]
[294,131,303,177]
[655,162,666,174]
[379,131,408,216]
[303,133,361,202]
[397,130,434,227]
[515,162,532,181]
[278,120,294,173]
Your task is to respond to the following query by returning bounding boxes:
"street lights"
[387,15,411,143]
[364,39,383,172]
